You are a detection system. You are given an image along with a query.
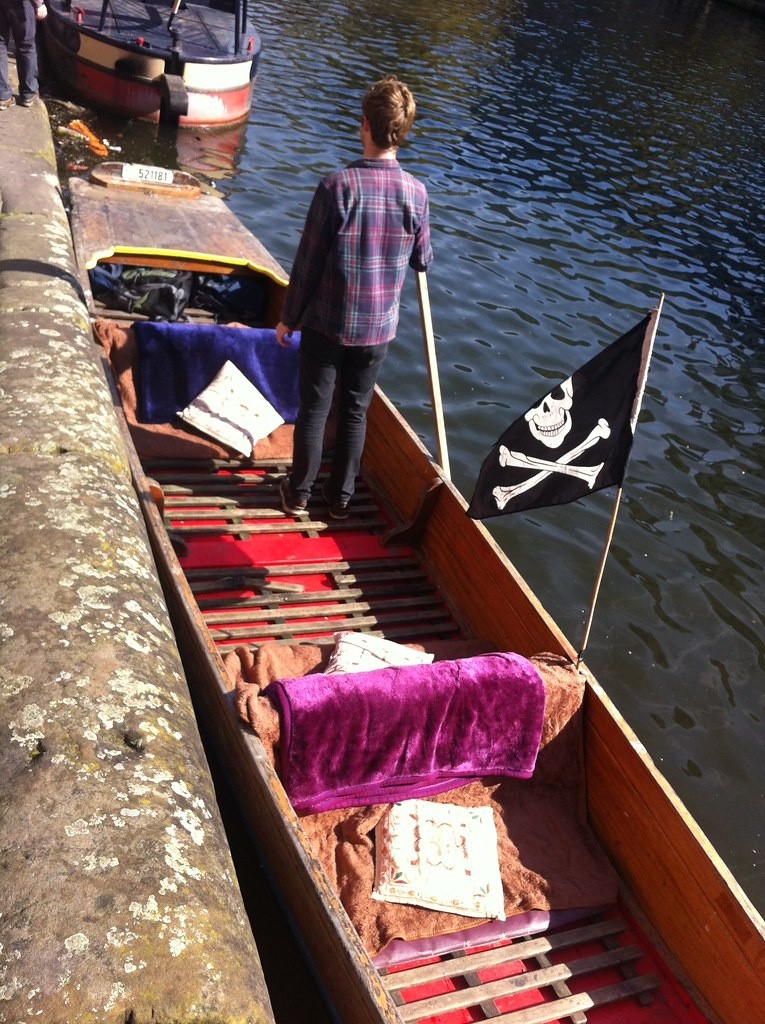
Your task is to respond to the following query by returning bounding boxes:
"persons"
[275,79,434,519]
[0,0,47,111]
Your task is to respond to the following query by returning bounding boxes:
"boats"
[41,0,263,128]
[67,162,764,1024]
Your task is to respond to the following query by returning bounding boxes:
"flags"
[465,310,661,520]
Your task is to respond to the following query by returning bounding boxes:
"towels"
[212,628,621,958]
[91,321,337,460]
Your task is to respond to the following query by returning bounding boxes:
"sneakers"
[21,90,39,106]
[0,96,16,111]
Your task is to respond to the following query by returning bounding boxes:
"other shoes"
[279,477,307,516]
[320,480,350,519]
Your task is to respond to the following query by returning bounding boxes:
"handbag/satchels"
[117,268,271,323]
